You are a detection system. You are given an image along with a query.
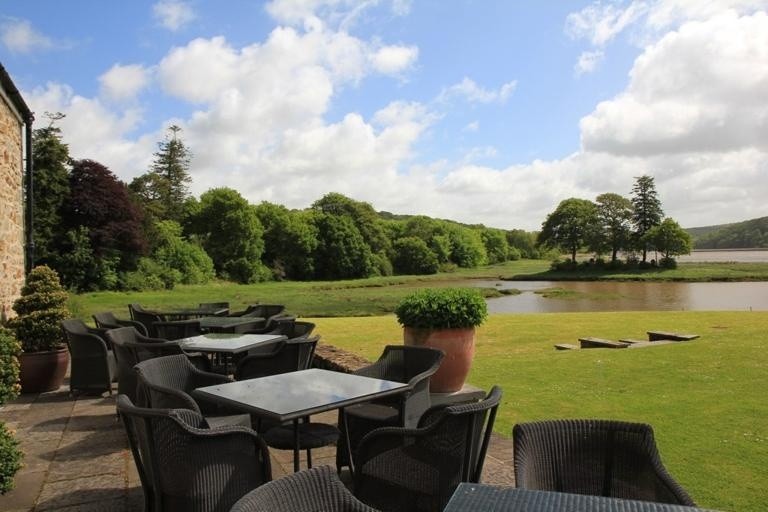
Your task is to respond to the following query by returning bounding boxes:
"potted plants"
[5,264,69,394]
[393,286,488,393]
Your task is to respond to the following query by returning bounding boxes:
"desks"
[192,367,409,476]
[163,332,288,376]
[441,480,718,512]
[190,316,265,334]
[157,306,229,318]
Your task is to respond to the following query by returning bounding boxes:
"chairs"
[353,386,502,509]
[133,353,252,431]
[229,464,381,512]
[121,340,203,370]
[152,320,208,339]
[336,345,446,475]
[248,321,315,343]
[127,302,166,329]
[116,394,273,512]
[105,326,168,373]
[244,313,295,334]
[61,318,121,395]
[92,311,149,341]
[235,304,286,331]
[235,334,321,379]
[512,418,697,508]
[200,302,230,317]
